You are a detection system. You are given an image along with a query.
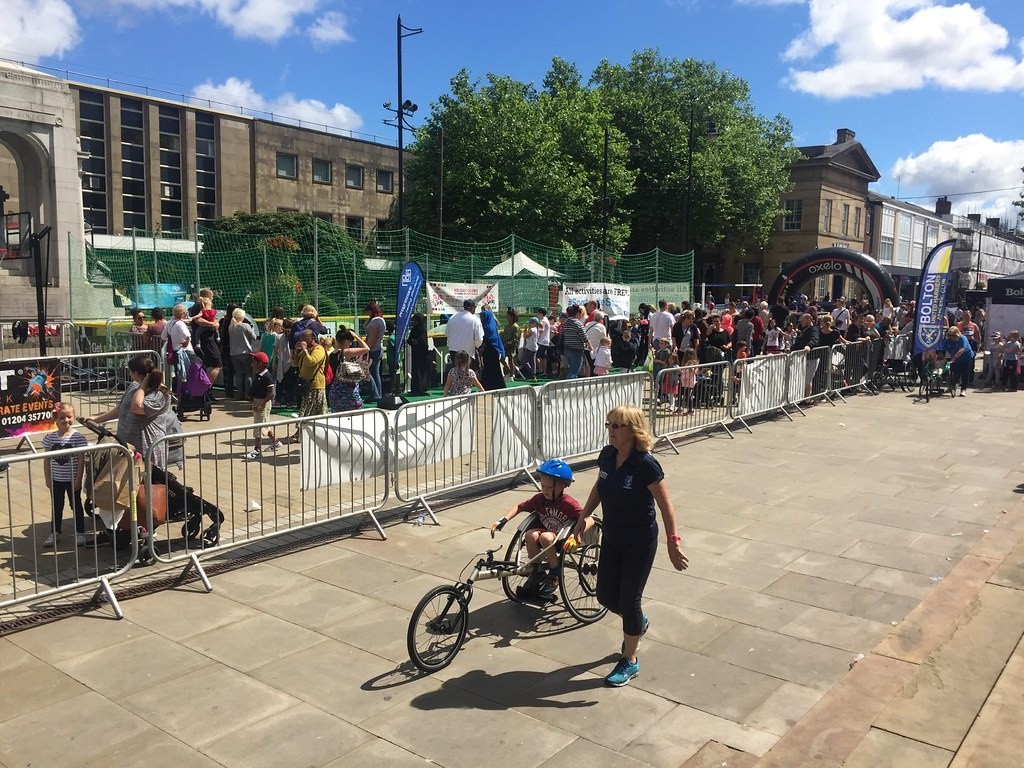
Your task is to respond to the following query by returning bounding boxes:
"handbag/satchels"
[550,335,564,354]
[90,450,168,530]
[335,350,371,385]
[642,349,654,373]
[324,363,334,385]
[296,377,312,395]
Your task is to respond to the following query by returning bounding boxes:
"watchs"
[668,535,681,543]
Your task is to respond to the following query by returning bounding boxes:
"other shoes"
[960,389,966,397]
[241,401,300,459]
[536,371,544,377]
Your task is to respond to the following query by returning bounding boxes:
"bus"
[63,311,539,383]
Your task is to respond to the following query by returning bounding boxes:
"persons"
[128,287,385,442]
[242,352,282,460]
[445,290,987,415]
[407,312,433,395]
[988,330,1024,392]
[574,406,690,687]
[84,355,171,547]
[491,458,594,596]
[444,351,484,397]
[42,400,88,547]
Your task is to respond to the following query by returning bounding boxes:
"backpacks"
[165,320,178,364]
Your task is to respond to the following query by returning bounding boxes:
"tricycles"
[406,506,609,674]
[919,357,956,402]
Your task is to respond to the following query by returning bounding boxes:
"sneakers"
[43,532,59,546]
[524,572,546,589]
[84,531,111,547]
[75,533,86,546]
[541,575,565,594]
[666,407,695,416]
[605,657,640,686]
[621,615,650,656]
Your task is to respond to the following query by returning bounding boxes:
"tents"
[983,271,1024,349]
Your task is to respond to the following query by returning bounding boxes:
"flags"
[913,239,956,355]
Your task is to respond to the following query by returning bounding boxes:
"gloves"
[490,517,507,539]
[563,532,578,555]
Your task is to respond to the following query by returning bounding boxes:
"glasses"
[948,335,954,337]
[605,421,625,429]
[137,316,146,320]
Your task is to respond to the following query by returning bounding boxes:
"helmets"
[536,458,577,482]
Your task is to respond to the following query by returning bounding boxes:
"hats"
[246,352,268,363]
[528,317,539,325]
[989,331,1001,338]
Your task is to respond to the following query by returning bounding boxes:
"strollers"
[76,415,227,569]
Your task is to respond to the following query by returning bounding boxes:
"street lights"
[602,122,640,251]
[685,92,720,251]
